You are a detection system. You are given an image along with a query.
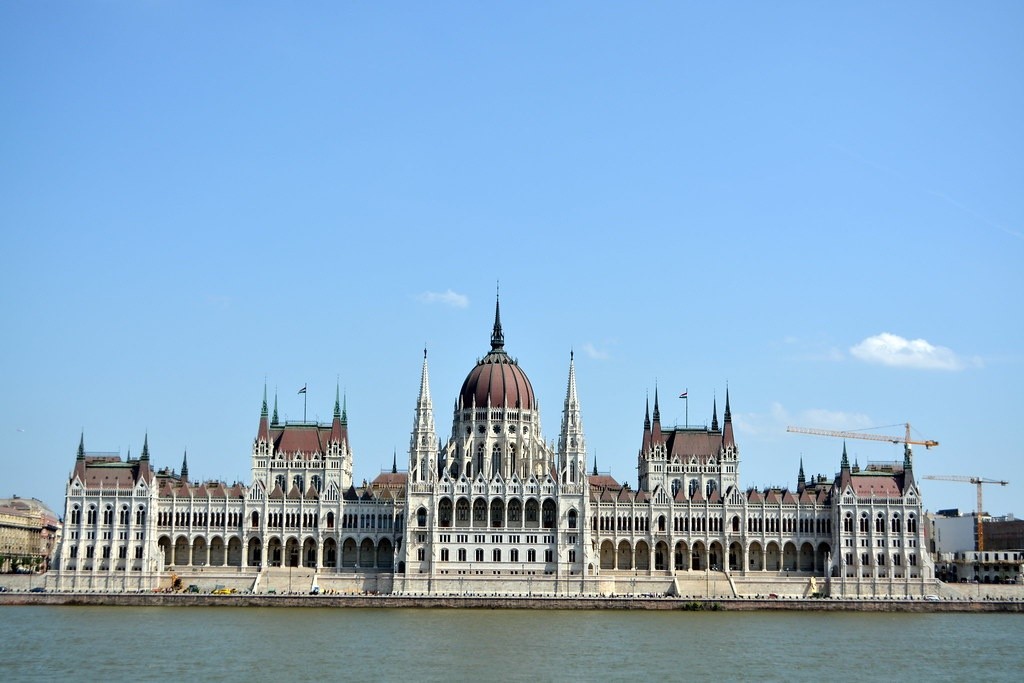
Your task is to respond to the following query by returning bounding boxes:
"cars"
[30,587,47,593]
[0,586,8,592]
[767,593,779,599]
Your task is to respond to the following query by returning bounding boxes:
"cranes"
[786,421,939,463]
[922,474,1009,551]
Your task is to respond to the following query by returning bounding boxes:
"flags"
[299,388,306,394]
[679,392,687,398]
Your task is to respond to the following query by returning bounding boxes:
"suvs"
[189,584,200,593]
[268,587,276,594]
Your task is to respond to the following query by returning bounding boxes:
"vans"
[211,588,230,594]
[926,595,939,600]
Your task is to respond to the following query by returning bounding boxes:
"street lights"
[674,566,677,576]
[597,564,598,575]
[201,562,204,571]
[355,563,357,573]
[259,562,261,571]
[786,567,789,577]
[636,567,638,576]
[315,563,318,573]
[395,563,397,573]
